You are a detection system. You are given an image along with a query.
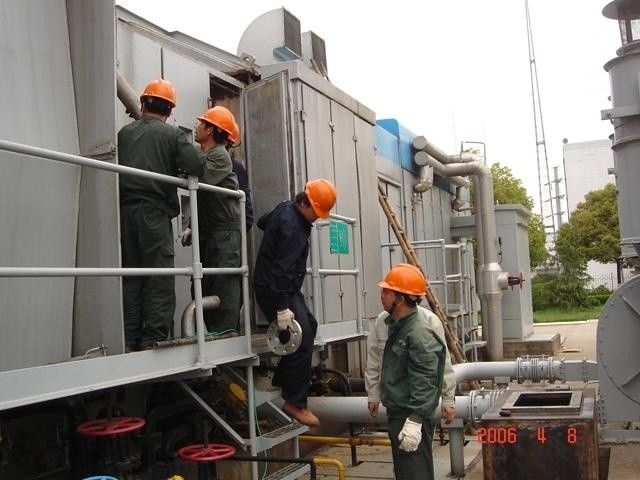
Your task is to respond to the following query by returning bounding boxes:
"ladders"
[378,179,482,391]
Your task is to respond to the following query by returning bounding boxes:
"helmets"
[306,179,337,218]
[196,106,239,144]
[378,264,426,296]
[140,80,176,107]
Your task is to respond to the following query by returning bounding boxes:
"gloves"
[277,309,295,332]
[398,419,422,451]
[180,228,193,247]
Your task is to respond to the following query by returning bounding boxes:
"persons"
[116,77,208,353]
[374,263,446,480]
[220,122,256,234]
[365,264,459,423]
[249,176,336,427]
[176,103,247,342]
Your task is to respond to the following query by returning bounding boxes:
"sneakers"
[281,402,321,427]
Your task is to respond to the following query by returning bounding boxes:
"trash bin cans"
[479,384,599,480]
